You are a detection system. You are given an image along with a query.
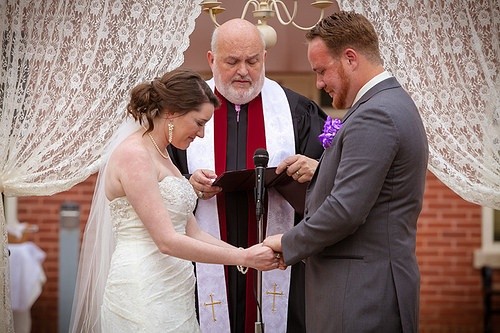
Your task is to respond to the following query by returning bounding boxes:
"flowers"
[317,114,343,150]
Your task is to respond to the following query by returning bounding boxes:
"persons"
[101,67,280,333]
[261,11,428,333]
[167,18,329,333]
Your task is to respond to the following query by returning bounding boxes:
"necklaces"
[140,120,170,159]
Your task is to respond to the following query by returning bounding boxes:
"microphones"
[253,148,270,220]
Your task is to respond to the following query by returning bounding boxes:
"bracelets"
[236,247,249,274]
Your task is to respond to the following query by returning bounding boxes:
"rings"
[198,191,203,198]
[296,171,301,177]
[277,253,280,258]
